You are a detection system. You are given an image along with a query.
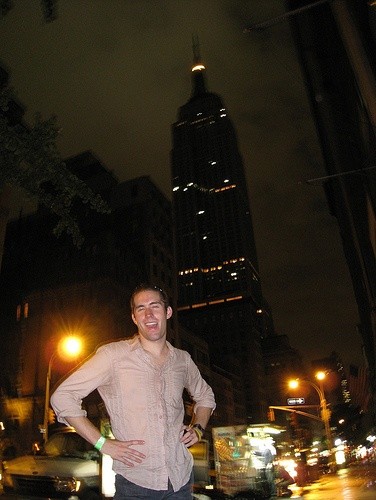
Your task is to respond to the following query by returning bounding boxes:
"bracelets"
[188,423,207,442]
[94,436,107,452]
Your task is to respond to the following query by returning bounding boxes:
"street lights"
[289,372,332,450]
[42,335,82,445]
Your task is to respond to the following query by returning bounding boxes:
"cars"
[3,429,101,500]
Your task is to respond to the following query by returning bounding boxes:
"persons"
[50,282,216,500]
[276,465,295,497]
